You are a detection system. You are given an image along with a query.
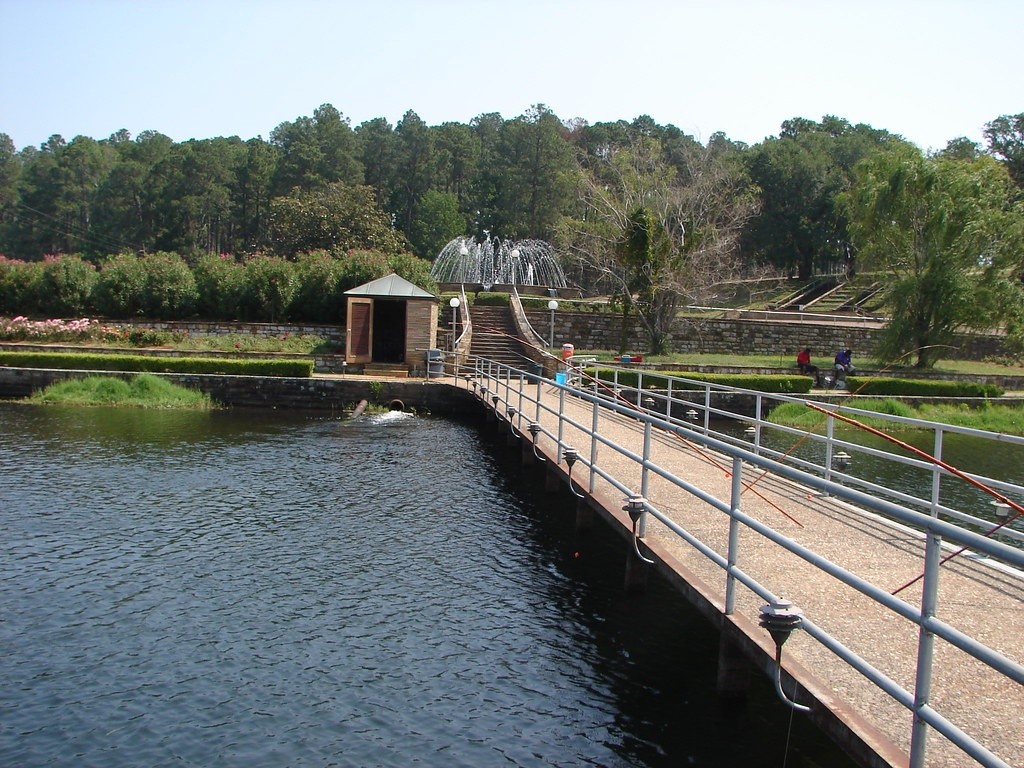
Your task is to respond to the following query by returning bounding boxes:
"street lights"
[546,299,559,355]
[460,248,468,286]
[449,297,460,357]
[512,249,521,288]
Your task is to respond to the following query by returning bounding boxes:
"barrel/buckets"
[557,372,567,387]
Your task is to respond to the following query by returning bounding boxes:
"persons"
[797,348,820,385]
[834,350,855,385]
